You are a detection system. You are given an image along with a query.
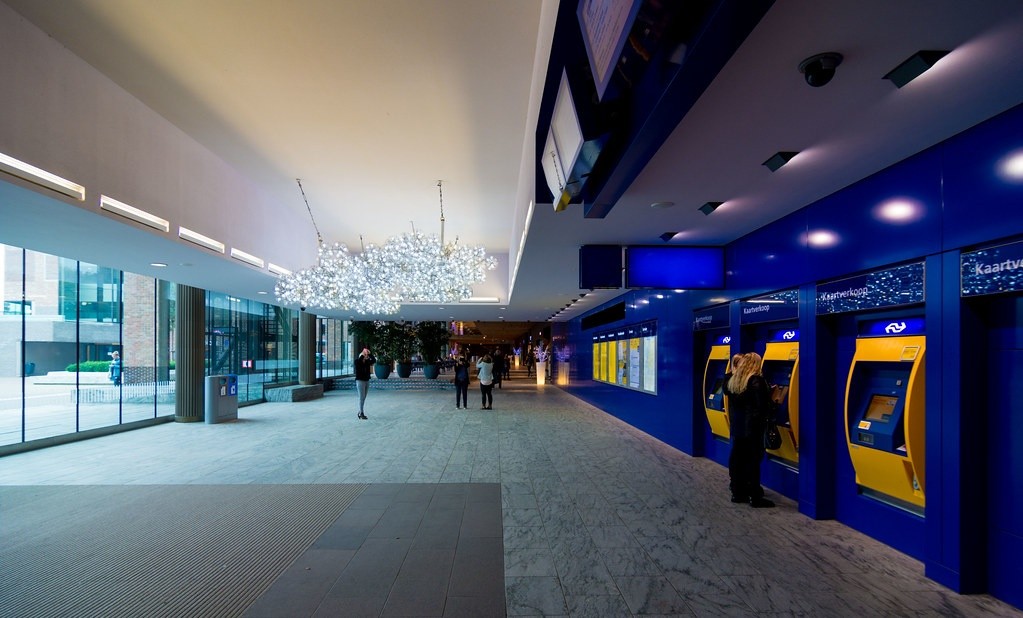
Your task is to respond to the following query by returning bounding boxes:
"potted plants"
[370,325,397,379]
[392,330,419,379]
[413,320,452,380]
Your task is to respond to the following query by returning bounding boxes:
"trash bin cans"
[25,362,35,374]
[205,374,241,424]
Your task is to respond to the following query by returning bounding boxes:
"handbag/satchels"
[765,420,782,450]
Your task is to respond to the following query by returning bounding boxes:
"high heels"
[358,413,367,419]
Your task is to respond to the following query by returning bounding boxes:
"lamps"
[272,178,499,315]
[761,151,801,173]
[697,202,724,215]
[881,49,954,88]
[659,232,678,242]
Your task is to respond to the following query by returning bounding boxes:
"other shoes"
[455,407,458,410]
[463,407,466,410]
[750,498,774,508]
[480,407,485,410]
[486,407,492,410]
[731,494,752,503]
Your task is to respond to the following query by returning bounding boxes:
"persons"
[492,349,510,390]
[354,347,376,420]
[454,356,470,409]
[107,351,124,387]
[524,349,534,377]
[476,355,494,410]
[722,352,779,508]
